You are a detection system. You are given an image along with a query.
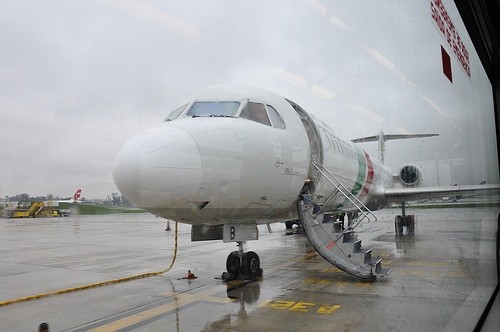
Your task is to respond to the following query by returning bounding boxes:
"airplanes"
[443,179,487,201]
[111,83,500,283]
